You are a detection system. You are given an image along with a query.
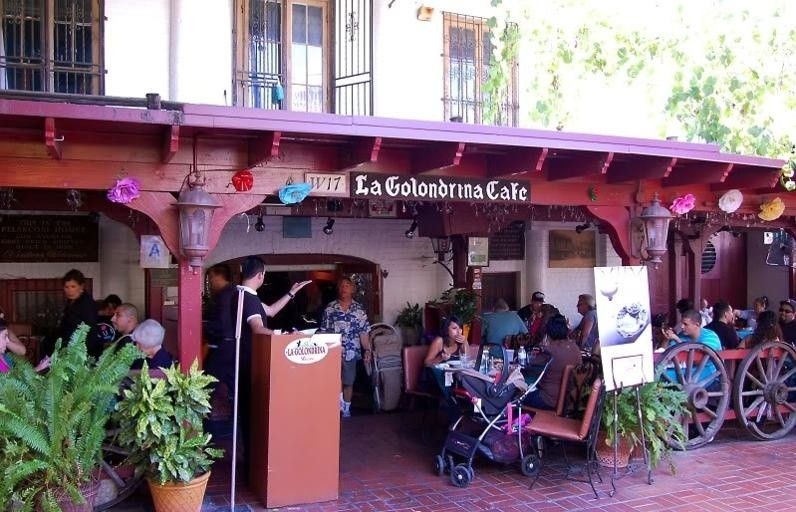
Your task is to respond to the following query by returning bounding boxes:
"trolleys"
[361,320,403,414]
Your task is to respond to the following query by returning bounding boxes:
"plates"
[743,327,753,331]
[448,360,460,366]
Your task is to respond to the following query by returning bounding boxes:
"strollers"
[434,342,556,486]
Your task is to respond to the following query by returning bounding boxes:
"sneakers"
[339,400,345,416]
[344,410,351,418]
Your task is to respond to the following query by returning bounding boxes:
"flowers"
[718,188,744,213]
[669,191,697,215]
[279,176,313,204]
[107,177,143,205]
[757,196,785,222]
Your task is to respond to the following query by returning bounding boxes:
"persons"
[421,316,470,427]
[229,255,314,457]
[204,262,240,403]
[514,314,584,411]
[0,294,177,387]
[478,291,599,366]
[648,294,796,416]
[316,275,372,420]
[61,269,97,348]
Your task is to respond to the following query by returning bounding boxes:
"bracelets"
[363,348,371,353]
[285,291,295,299]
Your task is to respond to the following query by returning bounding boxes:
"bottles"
[480,345,528,377]
[275,79,284,100]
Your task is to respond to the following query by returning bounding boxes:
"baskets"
[595,429,634,468]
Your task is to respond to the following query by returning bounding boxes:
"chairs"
[402,344,602,499]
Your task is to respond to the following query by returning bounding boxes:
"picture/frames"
[466,235,490,268]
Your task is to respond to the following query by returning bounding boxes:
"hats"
[780,299,796,308]
[532,292,545,303]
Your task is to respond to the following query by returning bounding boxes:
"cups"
[661,322,672,334]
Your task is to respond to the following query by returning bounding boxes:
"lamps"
[254,211,420,240]
[169,169,224,276]
[631,190,677,271]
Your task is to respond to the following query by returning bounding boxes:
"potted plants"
[392,299,426,346]
[578,363,690,477]
[448,289,479,340]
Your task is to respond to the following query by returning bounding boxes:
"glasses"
[778,308,794,313]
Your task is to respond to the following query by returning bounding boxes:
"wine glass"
[457,345,471,368]
